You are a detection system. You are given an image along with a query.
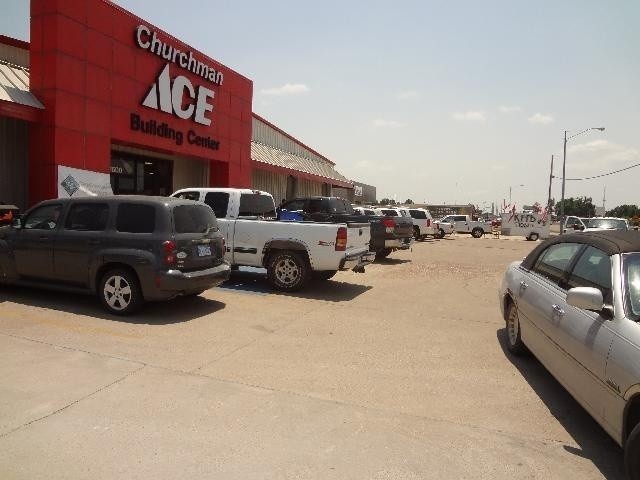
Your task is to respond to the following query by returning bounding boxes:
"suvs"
[355,204,433,240]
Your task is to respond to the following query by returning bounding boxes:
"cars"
[492,218,501,226]
[496,216,639,480]
[563,215,631,233]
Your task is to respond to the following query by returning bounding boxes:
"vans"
[0,193,235,314]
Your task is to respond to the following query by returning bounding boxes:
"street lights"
[509,183,526,203]
[559,126,608,236]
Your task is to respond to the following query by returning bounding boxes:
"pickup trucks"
[277,194,415,264]
[432,222,454,238]
[165,187,378,293]
[436,214,493,238]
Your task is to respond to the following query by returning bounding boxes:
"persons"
[282,198,286,204]
[632,216,639,231]
